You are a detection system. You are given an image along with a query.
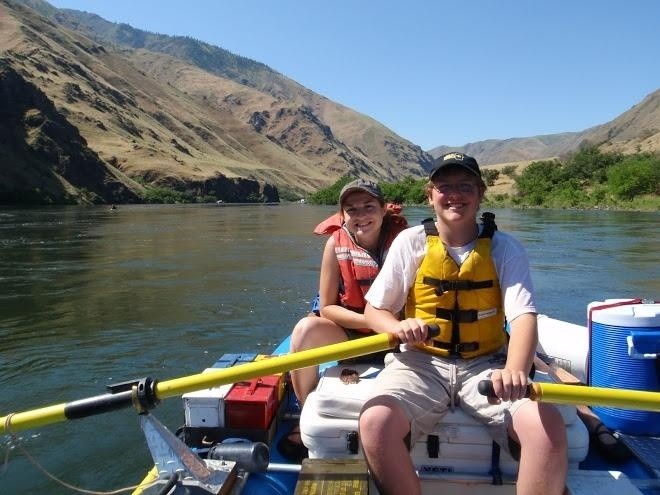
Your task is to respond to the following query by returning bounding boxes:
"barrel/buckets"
[587,298,659,434]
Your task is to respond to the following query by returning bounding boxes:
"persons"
[356,151,569,495]
[276,176,408,462]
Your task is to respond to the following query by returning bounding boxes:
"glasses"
[430,181,482,192]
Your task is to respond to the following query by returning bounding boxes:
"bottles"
[207,441,270,474]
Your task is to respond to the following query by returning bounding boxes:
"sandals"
[592,422,629,465]
[276,421,306,461]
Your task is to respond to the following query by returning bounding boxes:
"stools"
[299,363,589,477]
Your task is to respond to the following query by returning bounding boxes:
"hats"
[429,152,482,181]
[338,178,383,205]
